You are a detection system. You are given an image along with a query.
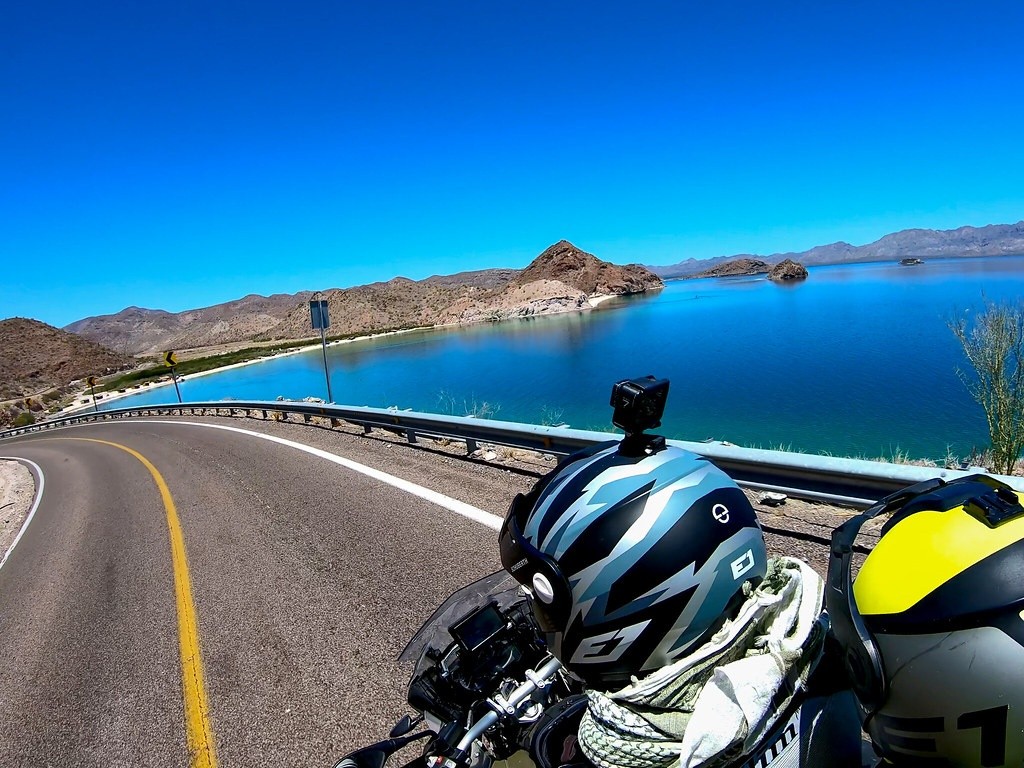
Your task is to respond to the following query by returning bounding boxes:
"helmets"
[852,490,1024,768]
[497,437,772,689]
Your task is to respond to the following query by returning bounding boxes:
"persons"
[485,376,1024,768]
